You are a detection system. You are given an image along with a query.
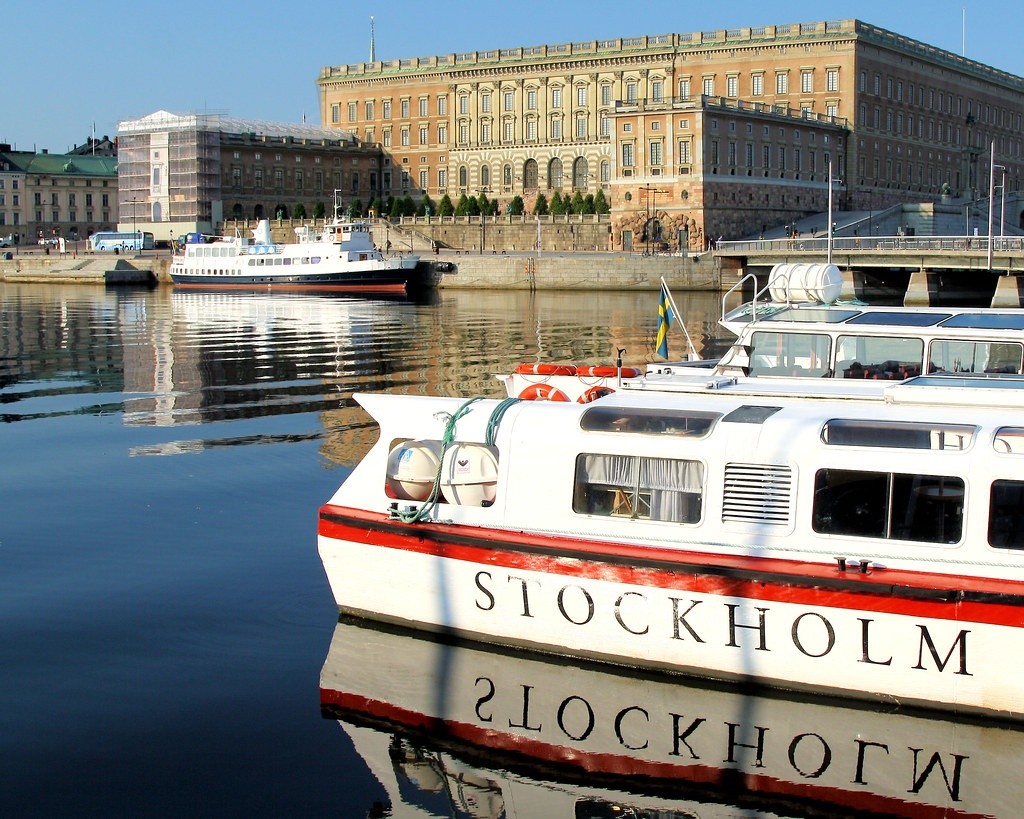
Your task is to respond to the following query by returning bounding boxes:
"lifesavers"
[577,365,641,377]
[578,387,615,404]
[516,364,575,376]
[519,384,570,401]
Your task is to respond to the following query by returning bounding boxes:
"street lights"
[638,183,657,252]
[124,197,145,250]
[475,187,494,255]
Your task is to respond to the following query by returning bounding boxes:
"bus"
[89,231,155,251]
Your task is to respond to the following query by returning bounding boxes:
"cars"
[38,236,61,245]
[0,237,13,248]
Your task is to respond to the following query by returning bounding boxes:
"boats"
[315,140,1023,819]
[169,188,422,294]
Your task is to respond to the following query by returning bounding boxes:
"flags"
[655,281,675,360]
